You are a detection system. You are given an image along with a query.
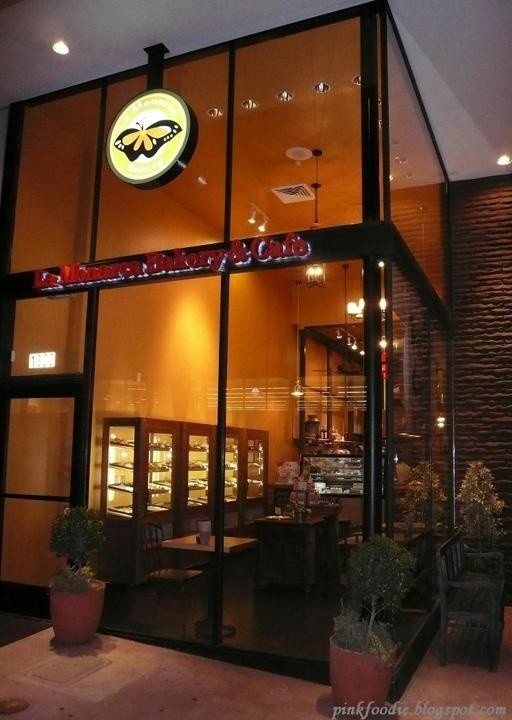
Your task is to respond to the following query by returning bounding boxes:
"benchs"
[435,524,505,673]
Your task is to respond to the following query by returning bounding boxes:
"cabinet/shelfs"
[300,452,387,525]
[101,415,268,588]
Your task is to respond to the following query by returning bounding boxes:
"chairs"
[137,521,204,641]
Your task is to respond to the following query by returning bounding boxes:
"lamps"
[290,280,304,398]
[246,201,270,233]
[303,147,327,291]
[334,326,358,351]
[347,265,387,320]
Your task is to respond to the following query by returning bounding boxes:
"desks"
[159,531,262,642]
[257,507,337,604]
[336,520,442,564]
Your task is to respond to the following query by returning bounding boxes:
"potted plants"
[326,534,418,720]
[44,505,108,646]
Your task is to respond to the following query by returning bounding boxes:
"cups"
[196,519,212,545]
